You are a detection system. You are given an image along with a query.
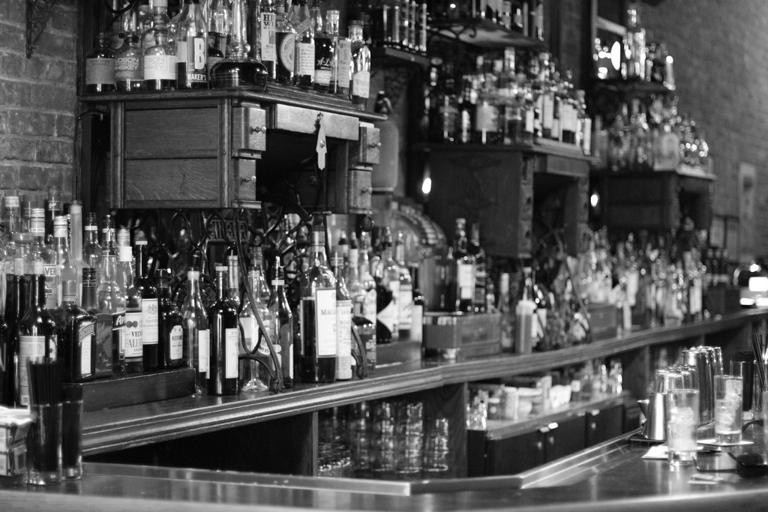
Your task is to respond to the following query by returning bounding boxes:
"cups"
[466,402,488,430]
[488,385,544,420]
[480,1,524,34]
[316,396,459,476]
[667,359,768,466]
[24,399,85,486]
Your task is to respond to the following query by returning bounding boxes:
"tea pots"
[635,346,725,440]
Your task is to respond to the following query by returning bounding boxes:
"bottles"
[84,1,376,106]
[594,7,681,95]
[435,46,586,144]
[572,359,623,395]
[0,196,493,408]
[497,216,730,356]
[605,96,709,175]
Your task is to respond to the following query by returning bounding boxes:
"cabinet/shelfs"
[581,393,645,451]
[424,142,595,263]
[465,410,581,480]
[91,82,388,226]
[597,163,720,259]
